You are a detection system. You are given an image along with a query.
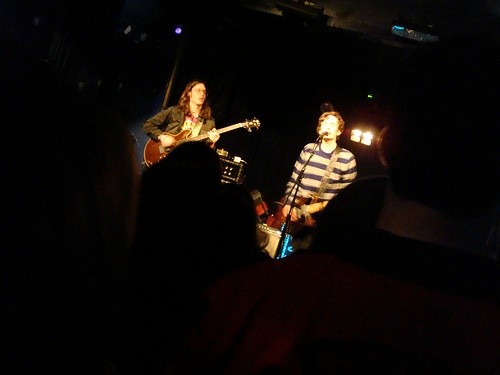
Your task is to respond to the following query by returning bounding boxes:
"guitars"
[266,195,329,229]
[143,117,261,168]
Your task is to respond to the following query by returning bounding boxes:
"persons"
[143,81,220,150]
[272,111,357,233]
[0,106,499,374]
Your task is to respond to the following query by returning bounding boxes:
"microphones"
[316,131,329,142]
